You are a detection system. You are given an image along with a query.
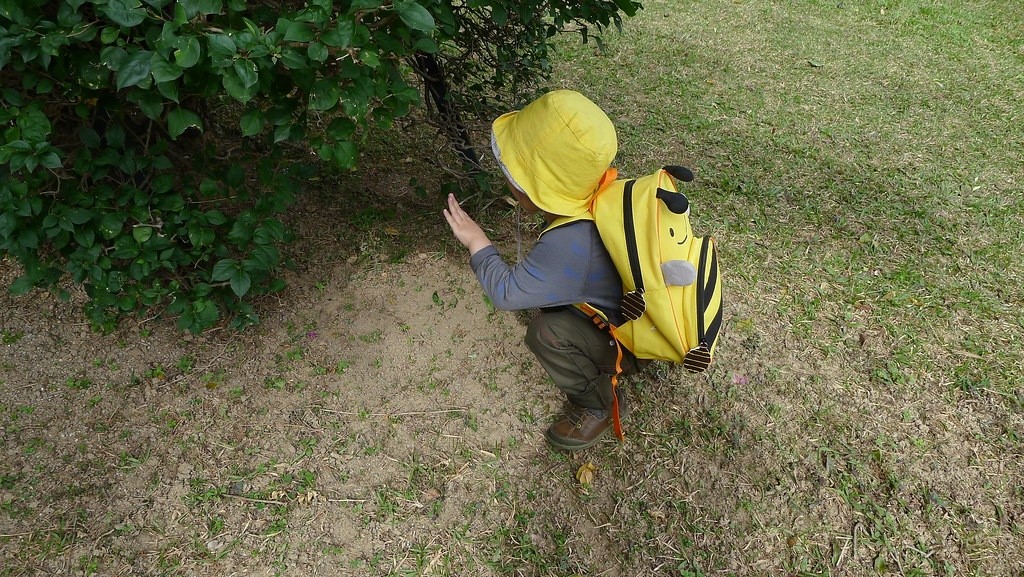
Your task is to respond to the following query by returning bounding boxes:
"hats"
[490,90,618,217]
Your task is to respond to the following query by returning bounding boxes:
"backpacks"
[535,165,723,375]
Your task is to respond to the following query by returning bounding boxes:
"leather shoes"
[546,385,632,451]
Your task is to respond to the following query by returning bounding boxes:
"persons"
[444,91,654,449]
